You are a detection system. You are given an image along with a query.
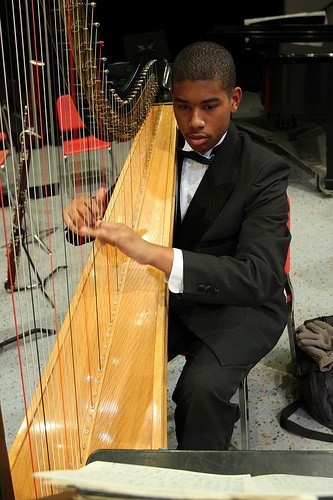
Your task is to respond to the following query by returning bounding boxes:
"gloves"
[296,320,333,372]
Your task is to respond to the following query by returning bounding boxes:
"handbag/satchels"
[278,313,332,441]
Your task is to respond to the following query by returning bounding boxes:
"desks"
[80,447,333,500]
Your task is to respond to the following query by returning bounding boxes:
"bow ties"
[176,147,213,165]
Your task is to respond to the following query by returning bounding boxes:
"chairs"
[56,94,113,200]
[239,196,299,451]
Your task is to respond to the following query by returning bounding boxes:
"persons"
[64,41,293,451]
